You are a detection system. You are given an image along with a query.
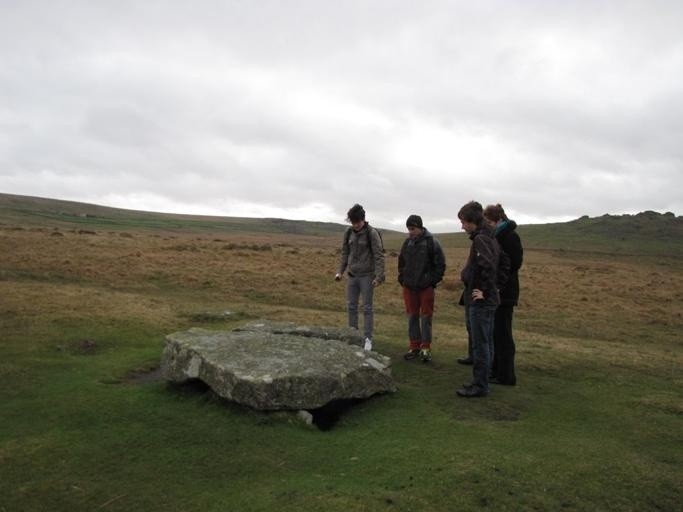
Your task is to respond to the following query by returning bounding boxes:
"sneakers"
[406,347,432,361]
[364,338,372,351]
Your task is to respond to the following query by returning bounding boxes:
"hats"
[406,215,423,228]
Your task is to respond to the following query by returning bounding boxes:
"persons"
[335,204,384,351]
[398,215,446,361]
[457,201,523,398]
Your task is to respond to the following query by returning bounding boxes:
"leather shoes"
[458,358,472,366]
[457,379,489,398]
